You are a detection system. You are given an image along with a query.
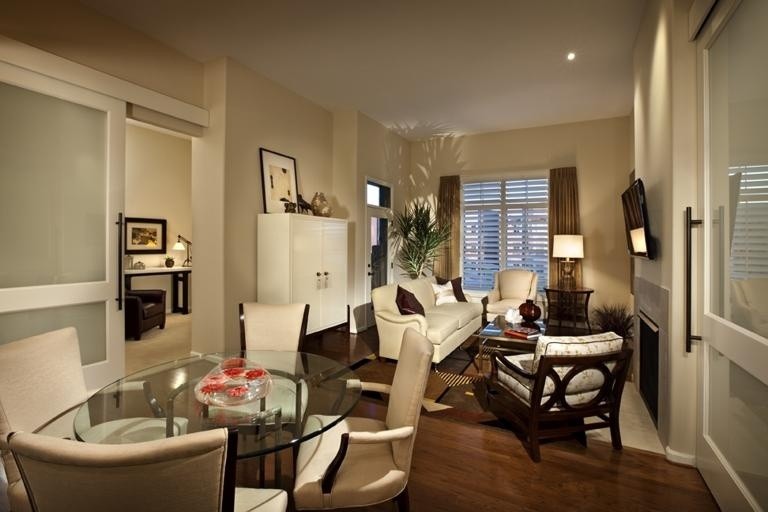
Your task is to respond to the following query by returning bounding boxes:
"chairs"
[237,303,308,485]
[5,426,291,511]
[295,325,435,511]
[0,325,190,446]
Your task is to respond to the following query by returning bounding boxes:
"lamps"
[173,233,190,269]
[552,234,586,286]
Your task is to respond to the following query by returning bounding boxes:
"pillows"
[396,285,430,315]
[435,277,468,302]
[431,281,458,306]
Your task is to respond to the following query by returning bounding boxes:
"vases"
[518,299,540,324]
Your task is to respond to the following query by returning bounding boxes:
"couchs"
[485,268,535,323]
[124,287,166,339]
[369,275,482,371]
[485,332,638,463]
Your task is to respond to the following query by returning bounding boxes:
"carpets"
[313,333,665,456]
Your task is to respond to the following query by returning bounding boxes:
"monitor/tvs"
[621,178,651,256]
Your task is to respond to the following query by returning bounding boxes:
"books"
[512,326,538,334]
[504,325,543,341]
[485,320,502,333]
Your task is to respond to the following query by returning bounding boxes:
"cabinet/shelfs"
[257,213,349,336]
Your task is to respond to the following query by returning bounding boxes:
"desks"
[544,286,591,334]
[70,347,363,510]
[124,265,191,315]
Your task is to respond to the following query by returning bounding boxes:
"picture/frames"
[123,217,167,256]
[257,146,300,214]
